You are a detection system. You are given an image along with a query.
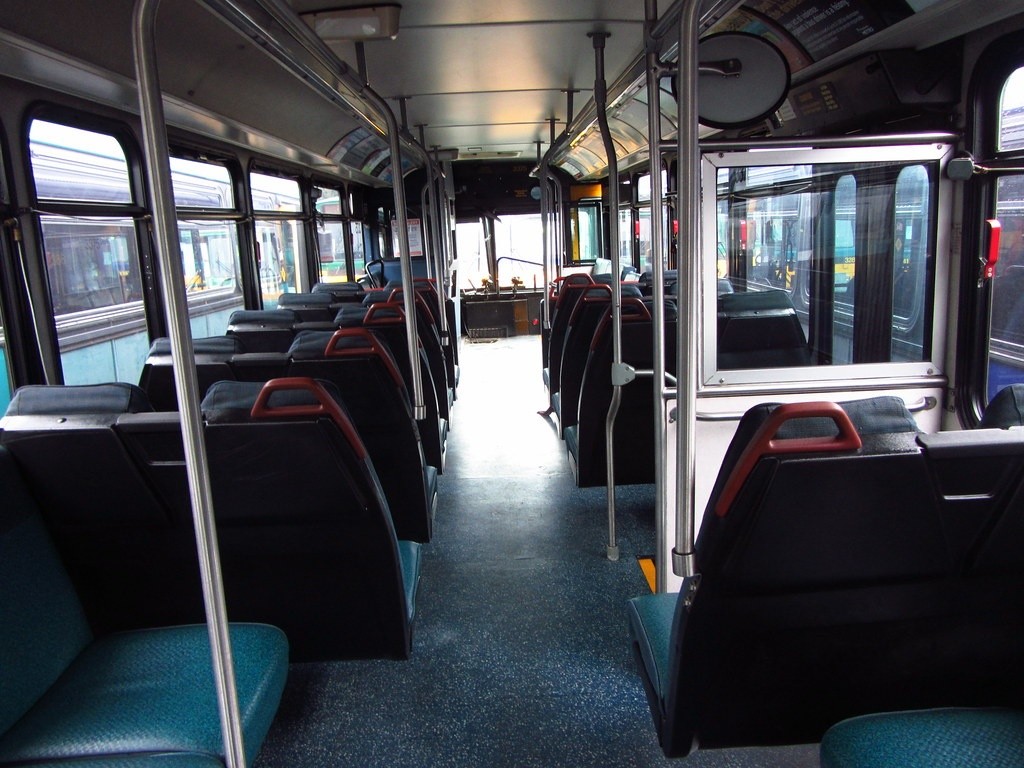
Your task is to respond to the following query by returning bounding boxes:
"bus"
[0,137,366,422]
[607,105,1024,414]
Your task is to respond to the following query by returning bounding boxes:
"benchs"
[0,258,460,768]
[538,258,1024,768]
